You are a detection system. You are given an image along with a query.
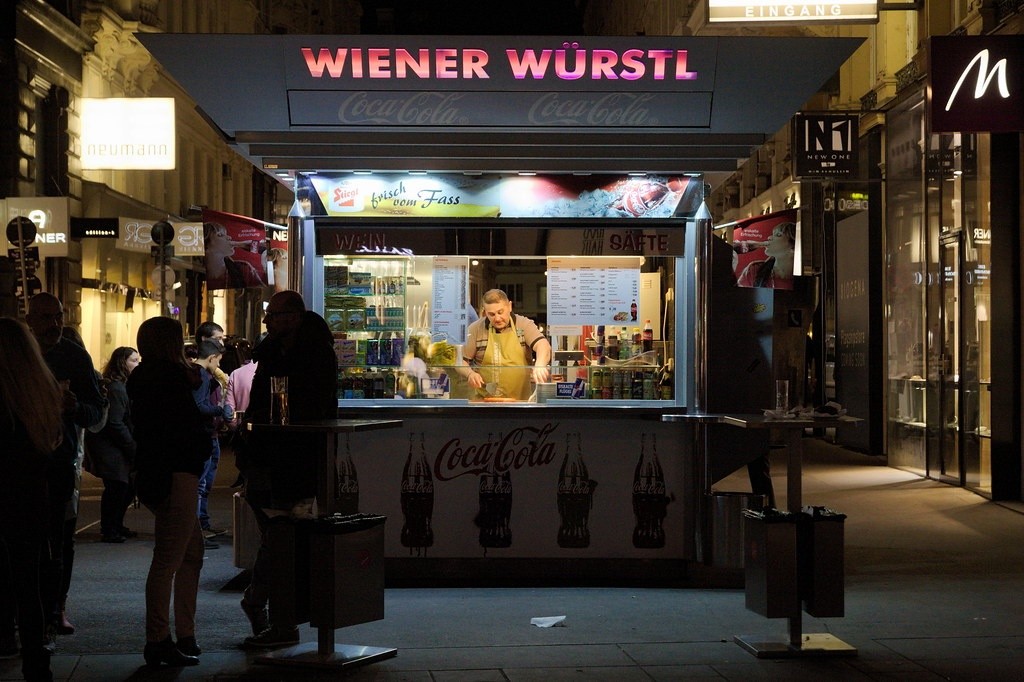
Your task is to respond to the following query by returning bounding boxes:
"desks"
[246,416,404,673]
[722,413,865,659]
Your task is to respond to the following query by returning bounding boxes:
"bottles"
[643,320,653,352]
[631,300,637,322]
[607,327,618,360]
[632,328,642,357]
[338,368,395,399]
[619,327,629,360]
[591,367,672,400]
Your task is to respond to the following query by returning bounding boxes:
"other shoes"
[209,526,228,536]
[120,530,137,537]
[202,530,215,539]
[101,533,127,543]
[202,539,219,549]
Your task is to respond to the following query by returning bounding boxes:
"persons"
[125,316,214,665]
[728,337,777,508]
[455,289,552,402]
[1,253,110,682]
[737,221,796,290]
[182,323,264,548]
[241,288,337,649]
[82,349,144,543]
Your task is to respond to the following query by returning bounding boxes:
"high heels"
[143,634,200,666]
[176,635,201,656]
[43,608,74,634]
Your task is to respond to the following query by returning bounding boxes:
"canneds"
[592,368,662,400]
[366,338,404,366]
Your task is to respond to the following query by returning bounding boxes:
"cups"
[776,379,788,417]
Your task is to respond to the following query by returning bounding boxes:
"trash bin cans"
[741,505,846,617]
[308,514,387,631]
[701,491,768,566]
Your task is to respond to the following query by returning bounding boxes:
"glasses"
[267,310,289,320]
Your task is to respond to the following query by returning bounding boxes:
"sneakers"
[244,627,301,647]
[240,597,271,634]
